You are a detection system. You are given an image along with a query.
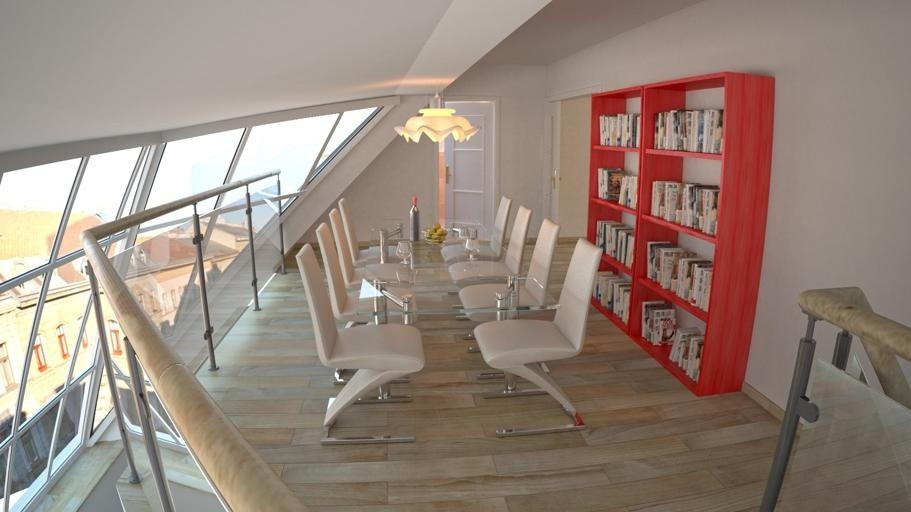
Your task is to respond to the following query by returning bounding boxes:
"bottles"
[409,195,420,243]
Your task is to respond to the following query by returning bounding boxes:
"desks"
[351,221,562,402]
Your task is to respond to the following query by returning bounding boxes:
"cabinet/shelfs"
[583,71,776,398]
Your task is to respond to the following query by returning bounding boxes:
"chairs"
[471,237,606,439]
[293,197,425,446]
[448,205,532,340]
[442,196,513,308]
[459,218,562,382]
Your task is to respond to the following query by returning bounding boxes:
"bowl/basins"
[422,224,452,244]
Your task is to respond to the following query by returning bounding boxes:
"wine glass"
[396,241,413,265]
[464,238,481,262]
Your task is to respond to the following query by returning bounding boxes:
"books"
[592,109,723,383]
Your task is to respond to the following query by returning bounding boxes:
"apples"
[427,223,447,241]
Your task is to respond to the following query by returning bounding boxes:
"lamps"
[393,90,481,144]
